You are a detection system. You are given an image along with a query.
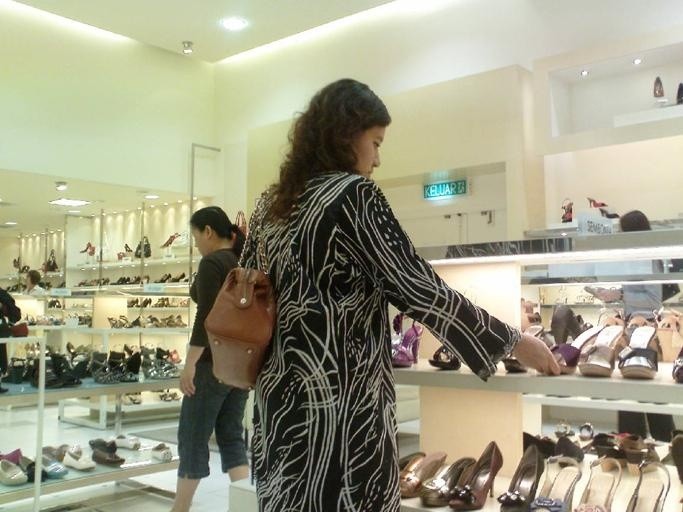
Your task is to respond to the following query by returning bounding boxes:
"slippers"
[584,286,621,302]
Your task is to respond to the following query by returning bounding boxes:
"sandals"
[555,422,593,441]
[521,298,542,331]
[399,441,503,511]
[598,306,683,329]
[498,444,670,512]
[503,325,657,377]
[428,345,461,370]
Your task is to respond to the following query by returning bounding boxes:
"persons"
[171,206,252,512]
[0,287,22,383]
[236,79,561,512]
[21,270,45,294]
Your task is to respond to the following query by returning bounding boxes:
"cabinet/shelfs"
[0,256,203,505]
[230,230,683,512]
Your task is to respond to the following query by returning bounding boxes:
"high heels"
[79,315,92,327]
[154,272,185,282]
[586,196,607,207]
[108,314,187,328]
[79,278,109,286]
[110,275,149,285]
[81,242,95,255]
[57,282,65,288]
[48,299,61,308]
[599,208,620,218]
[551,304,592,344]
[117,243,132,259]
[561,198,573,222]
[127,297,189,307]
[390,312,424,367]
[1,342,181,390]
[12,259,29,273]
[160,392,181,401]
[524,432,660,462]
[23,313,61,326]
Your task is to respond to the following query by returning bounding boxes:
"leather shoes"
[90,438,125,465]
[111,435,140,449]
[42,444,95,471]
[0,448,68,486]
[654,76,664,97]
[6,283,26,293]
[162,233,180,248]
[153,442,173,460]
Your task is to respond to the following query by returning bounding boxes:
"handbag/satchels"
[205,267,275,390]
[42,248,58,271]
[135,236,151,258]
[232,211,246,239]
[10,322,28,337]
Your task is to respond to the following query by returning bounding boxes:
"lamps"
[55,182,67,191]
[182,42,194,55]
